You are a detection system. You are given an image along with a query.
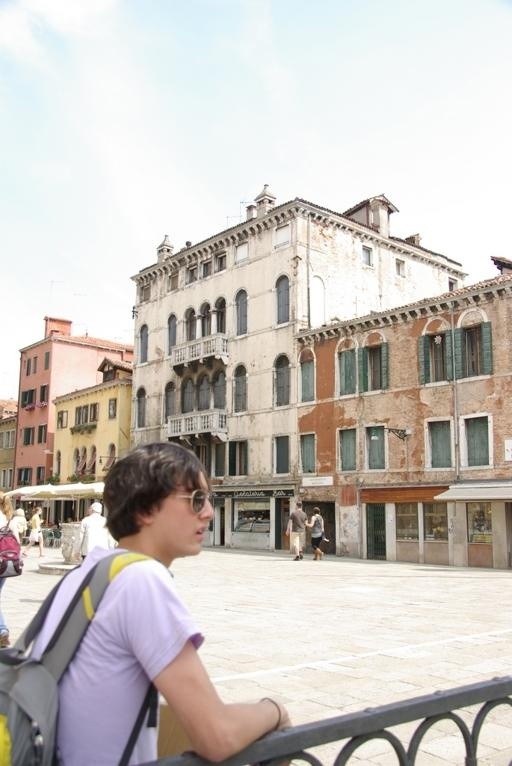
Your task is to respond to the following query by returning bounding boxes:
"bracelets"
[260,697,282,733]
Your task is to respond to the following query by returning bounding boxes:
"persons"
[22,507,46,558]
[0,489,24,649]
[74,502,119,562]
[305,506,328,560]
[0,438,292,766]
[285,500,308,561]
[9,507,28,549]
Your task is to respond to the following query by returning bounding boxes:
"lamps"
[371,427,410,440]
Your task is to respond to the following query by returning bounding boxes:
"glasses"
[165,488,217,517]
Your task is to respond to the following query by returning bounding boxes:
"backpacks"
[0,548,180,766]
[0,519,24,579]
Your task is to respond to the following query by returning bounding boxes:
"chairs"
[42,528,61,548]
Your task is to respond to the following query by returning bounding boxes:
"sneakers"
[22,551,46,558]
[293,550,324,561]
